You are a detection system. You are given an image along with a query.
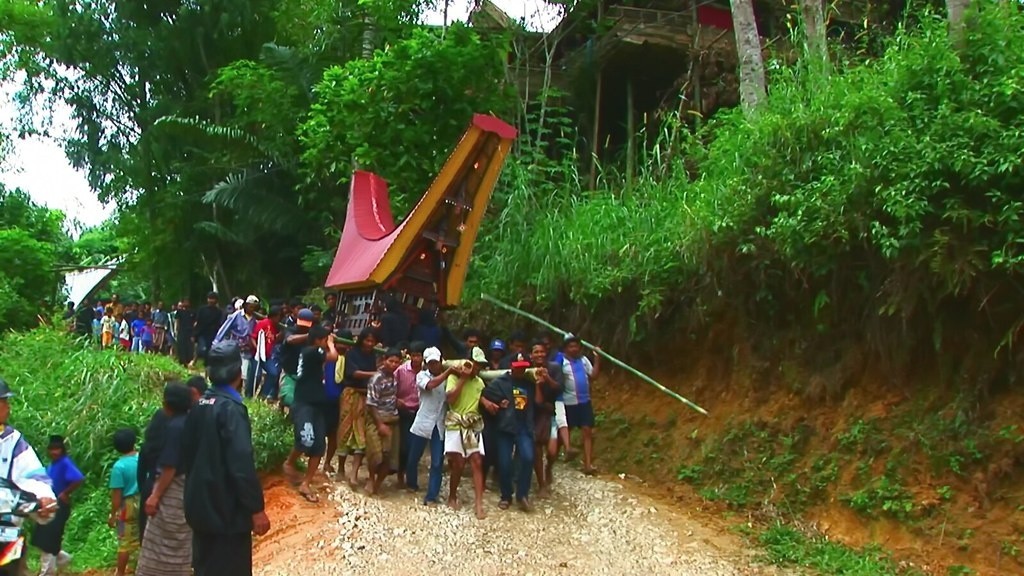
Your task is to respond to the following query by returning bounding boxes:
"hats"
[234,299,244,309]
[296,308,313,327]
[563,332,582,344]
[0,378,17,398]
[246,294,260,304]
[488,338,506,351]
[510,353,531,367]
[423,346,441,364]
[469,347,488,363]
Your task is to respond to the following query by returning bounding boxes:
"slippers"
[283,462,300,485]
[299,488,319,501]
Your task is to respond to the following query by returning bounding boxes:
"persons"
[0,292,601,576]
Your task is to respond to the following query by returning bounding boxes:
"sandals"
[498,499,512,510]
[517,498,532,511]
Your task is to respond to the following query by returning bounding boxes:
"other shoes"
[56,551,71,571]
[584,465,599,474]
[564,447,579,461]
